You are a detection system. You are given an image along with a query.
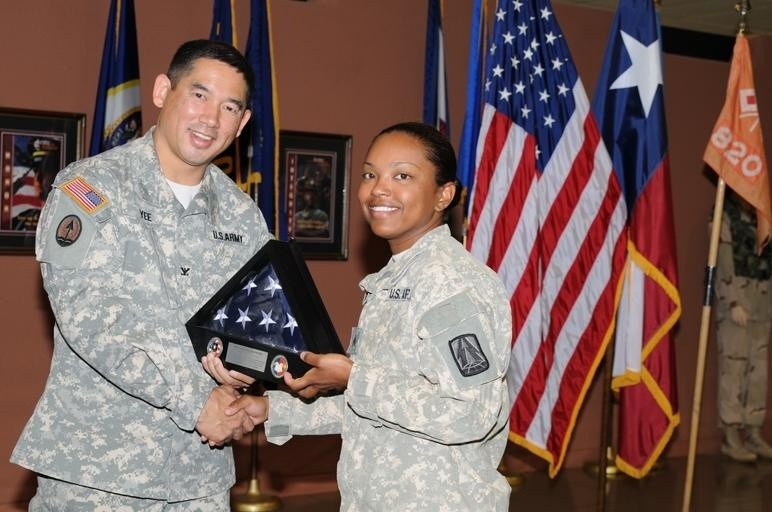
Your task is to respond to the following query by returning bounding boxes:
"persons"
[706,192,772,463]
[200,122,513,512]
[7,37,273,512]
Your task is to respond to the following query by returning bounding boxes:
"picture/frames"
[280,130,353,261]
[0,107,86,256]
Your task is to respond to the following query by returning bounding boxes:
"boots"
[719,423,756,462]
[744,422,772,460]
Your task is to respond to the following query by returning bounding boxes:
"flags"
[87,0,141,157]
[701,31,772,255]
[208,0,239,186]
[423,1,684,483]
[238,0,288,242]
